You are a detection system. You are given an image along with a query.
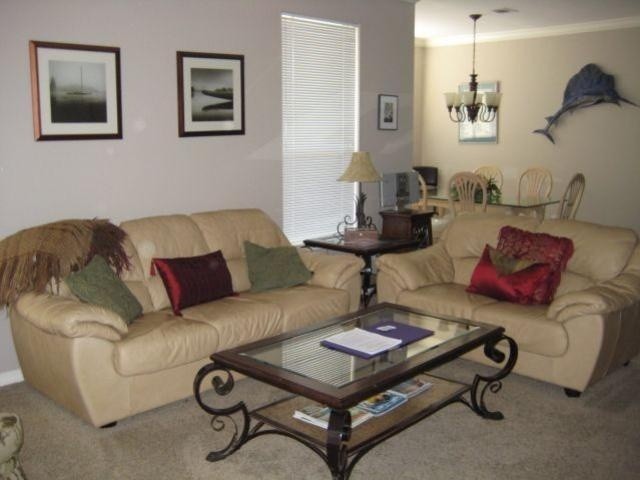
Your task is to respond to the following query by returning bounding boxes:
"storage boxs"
[376,207,435,240]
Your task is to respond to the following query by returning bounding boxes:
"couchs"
[1,207,364,429]
[370,212,639,398]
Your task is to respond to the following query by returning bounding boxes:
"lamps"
[442,13,501,122]
[335,151,383,238]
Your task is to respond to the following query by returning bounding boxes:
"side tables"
[301,232,420,308]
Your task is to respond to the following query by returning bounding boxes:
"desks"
[193,300,518,480]
[425,193,561,221]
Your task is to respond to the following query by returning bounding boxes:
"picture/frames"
[175,49,245,138]
[377,93,399,132]
[29,40,123,143]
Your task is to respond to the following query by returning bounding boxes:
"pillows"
[462,244,555,304]
[62,254,143,326]
[491,226,574,280]
[149,248,238,317]
[240,237,314,293]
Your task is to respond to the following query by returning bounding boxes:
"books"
[291,402,372,428]
[387,377,432,399]
[356,390,407,416]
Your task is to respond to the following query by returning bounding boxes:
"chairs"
[518,168,552,200]
[557,172,585,221]
[412,169,428,210]
[448,172,487,217]
[474,165,503,194]
